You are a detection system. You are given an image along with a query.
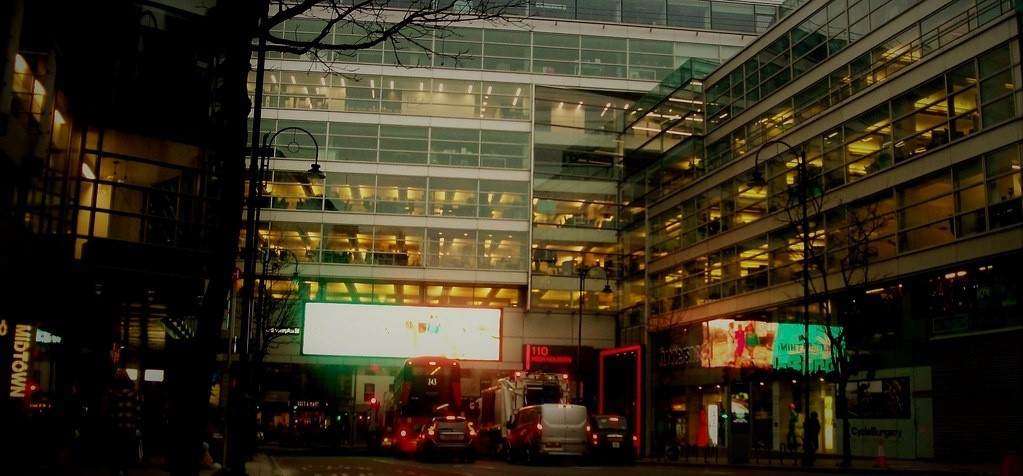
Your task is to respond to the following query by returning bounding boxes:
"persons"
[804,412,820,466]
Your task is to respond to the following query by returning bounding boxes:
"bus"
[389,356,462,428]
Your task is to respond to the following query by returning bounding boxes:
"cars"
[288,372,639,459]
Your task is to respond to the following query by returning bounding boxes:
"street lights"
[575,265,614,404]
[237,126,327,455]
[745,139,812,468]
[250,247,300,401]
